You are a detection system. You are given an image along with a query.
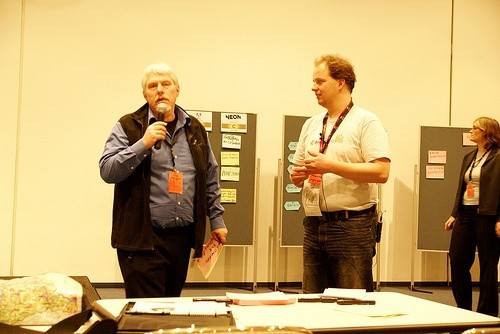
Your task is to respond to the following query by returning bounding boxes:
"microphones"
[154,103,167,149]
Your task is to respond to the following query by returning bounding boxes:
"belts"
[308,205,377,222]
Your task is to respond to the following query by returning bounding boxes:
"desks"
[97,292,500,334]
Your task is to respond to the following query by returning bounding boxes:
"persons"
[290,54,391,294]
[444,116,500,317]
[99,62,229,298]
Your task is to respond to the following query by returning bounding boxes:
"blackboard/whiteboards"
[185,110,257,246]
[281,115,310,247]
[417,126,477,252]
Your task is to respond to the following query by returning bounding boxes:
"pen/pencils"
[298,296,375,306]
[193,298,232,302]
